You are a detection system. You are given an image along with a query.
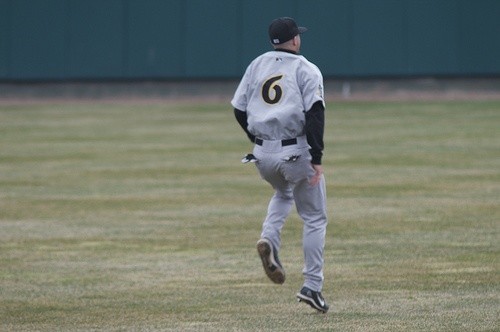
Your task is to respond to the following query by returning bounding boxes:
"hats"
[269,17,308,45]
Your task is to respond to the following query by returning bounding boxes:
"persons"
[229,16,330,313]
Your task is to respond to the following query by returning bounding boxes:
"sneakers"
[257,238,285,285]
[295,286,329,313]
